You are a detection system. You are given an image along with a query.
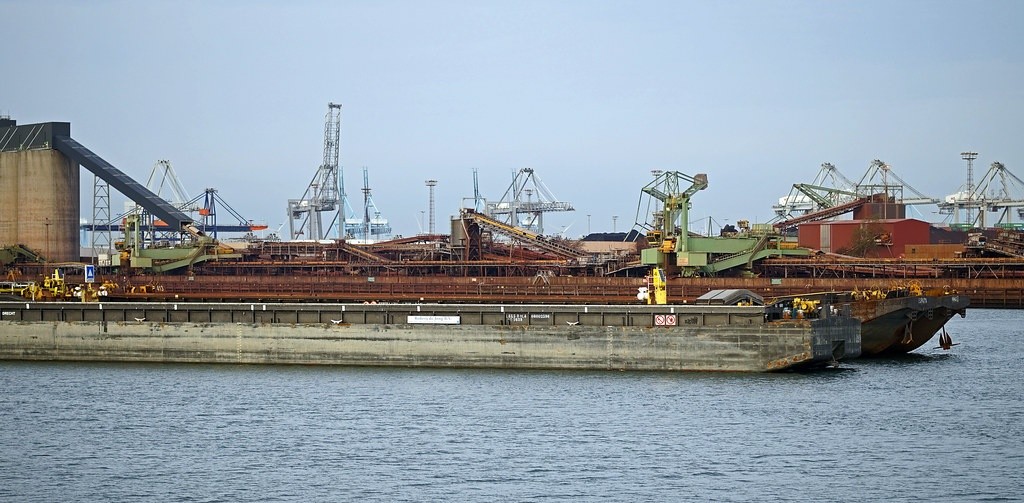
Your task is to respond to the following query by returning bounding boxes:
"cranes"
[779,160,1024,211]
[485,168,576,214]
[286,102,343,220]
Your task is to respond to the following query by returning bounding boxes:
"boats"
[0,207,971,374]
